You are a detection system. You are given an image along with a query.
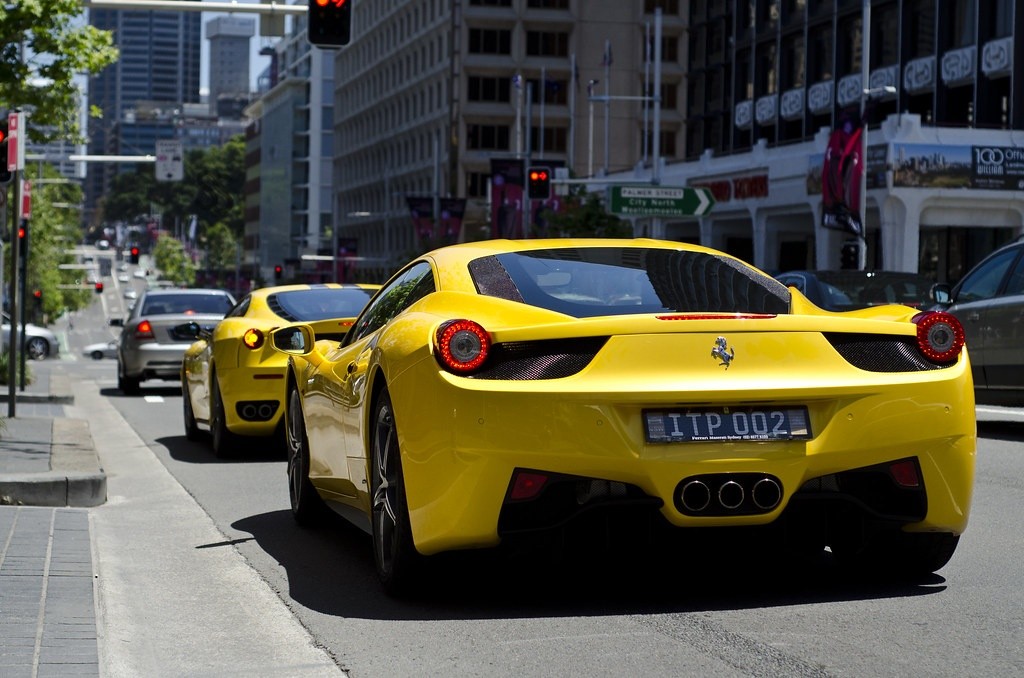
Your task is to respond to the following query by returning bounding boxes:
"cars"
[116,263,153,313]
[771,268,940,313]
[0,307,61,361]
[107,288,238,398]
[81,337,122,360]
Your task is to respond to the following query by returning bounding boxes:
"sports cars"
[172,281,389,462]
[266,238,978,606]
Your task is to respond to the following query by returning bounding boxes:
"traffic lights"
[308,0,356,53]
[94,282,104,294]
[130,245,140,265]
[524,165,552,201]
[18,216,28,260]
[274,263,284,286]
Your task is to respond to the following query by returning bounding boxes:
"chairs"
[147,305,165,314]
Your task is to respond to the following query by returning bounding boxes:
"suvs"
[917,237,1023,412]
